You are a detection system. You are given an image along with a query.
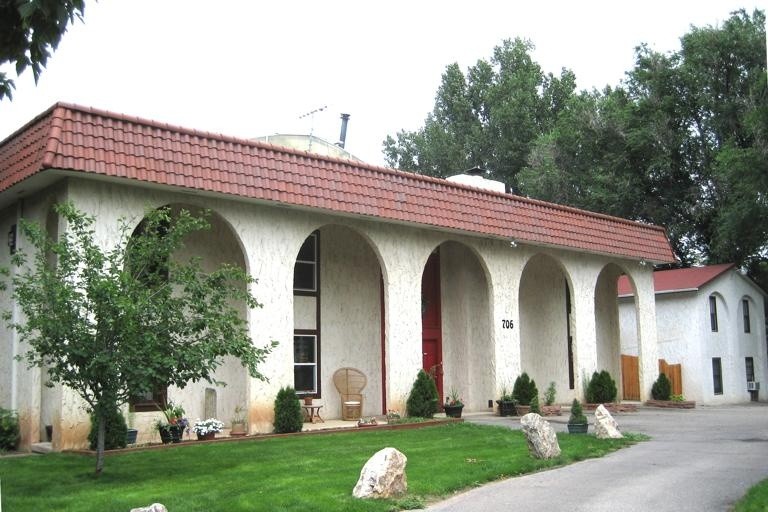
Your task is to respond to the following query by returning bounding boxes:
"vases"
[197,431,215,441]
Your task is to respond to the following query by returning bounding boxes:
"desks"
[302,404,324,424]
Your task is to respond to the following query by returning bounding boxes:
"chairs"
[341,393,362,421]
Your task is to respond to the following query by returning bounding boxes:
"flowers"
[184,417,225,437]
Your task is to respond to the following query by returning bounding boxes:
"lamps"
[510,241,517,248]
[639,259,646,265]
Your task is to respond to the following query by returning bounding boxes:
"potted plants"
[230,404,248,436]
[147,393,189,444]
[567,398,589,433]
[496,377,519,417]
[304,392,313,405]
[442,385,464,418]
[511,372,538,416]
[127,405,138,444]
